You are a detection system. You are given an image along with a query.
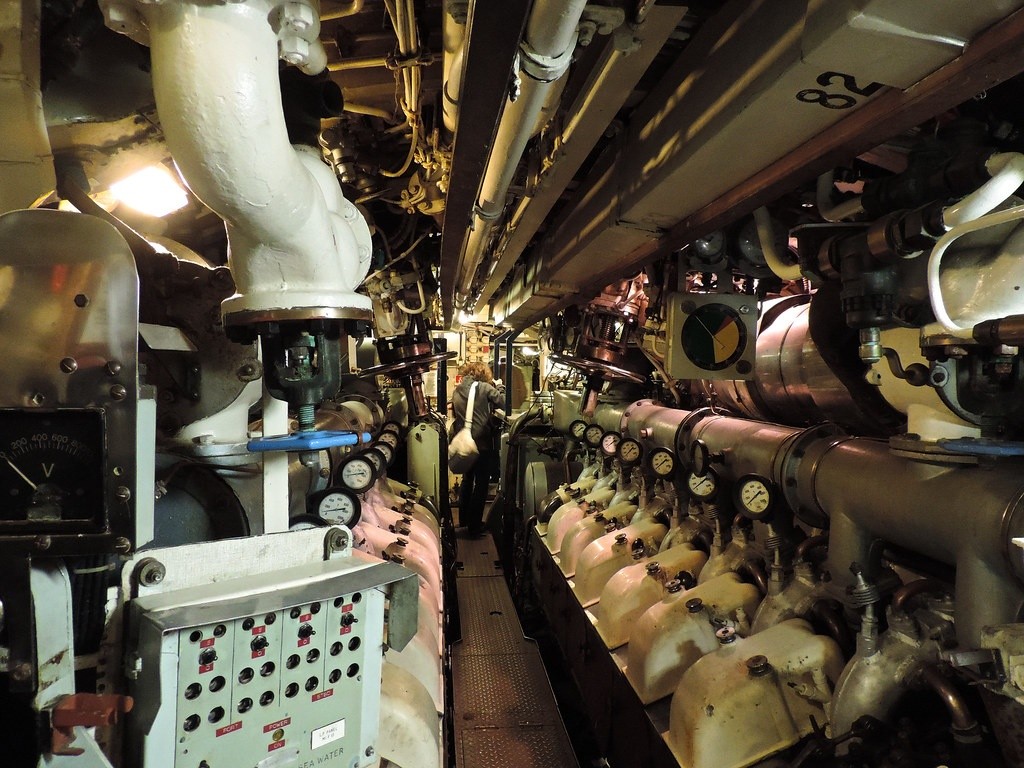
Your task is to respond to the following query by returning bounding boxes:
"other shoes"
[461,522,487,537]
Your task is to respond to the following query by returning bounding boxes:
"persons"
[455,360,508,537]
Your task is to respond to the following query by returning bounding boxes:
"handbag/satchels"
[448,427,479,474]
